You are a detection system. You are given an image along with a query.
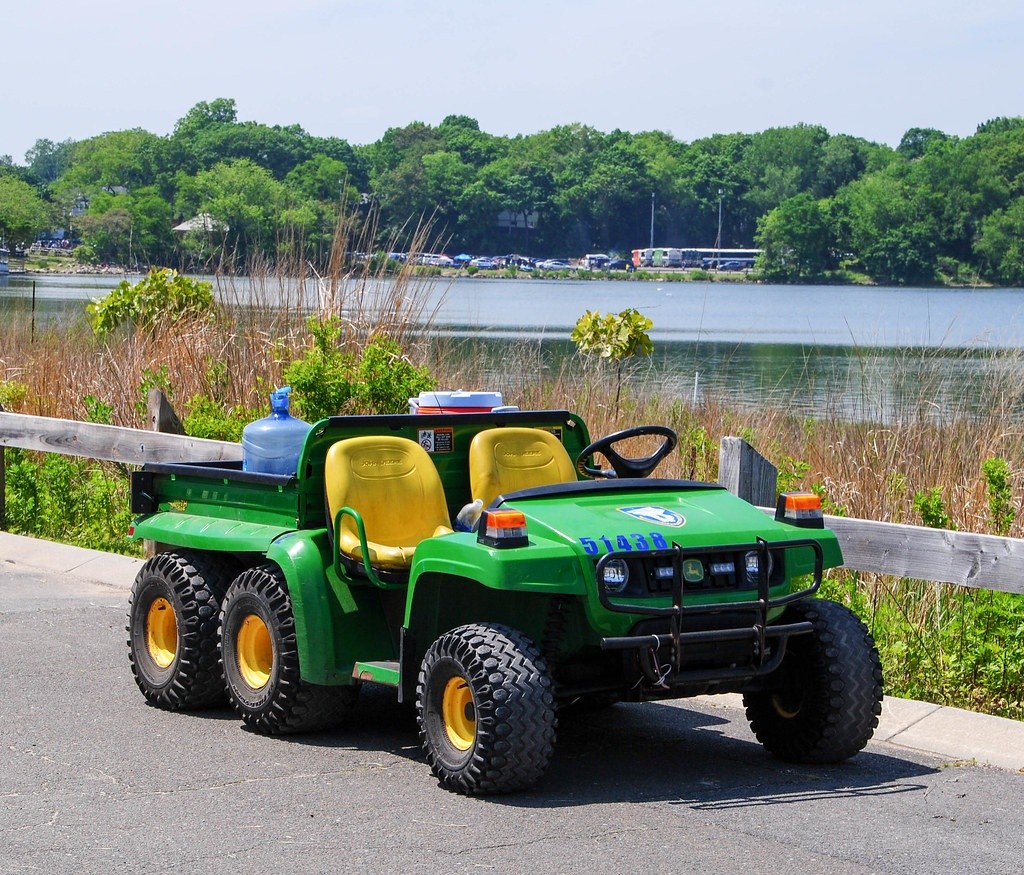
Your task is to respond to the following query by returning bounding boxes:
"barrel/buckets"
[409,390,519,416]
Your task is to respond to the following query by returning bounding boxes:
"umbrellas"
[455,254,471,269]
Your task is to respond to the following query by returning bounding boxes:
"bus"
[631,248,764,269]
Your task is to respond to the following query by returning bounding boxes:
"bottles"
[241,392,312,477]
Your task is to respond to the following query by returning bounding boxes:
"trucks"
[126,410,884,802]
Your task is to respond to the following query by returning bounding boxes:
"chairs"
[323,436,456,583]
[468,428,577,517]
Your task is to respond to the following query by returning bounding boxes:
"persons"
[509,255,536,272]
[626,263,630,273]
[680,259,686,271]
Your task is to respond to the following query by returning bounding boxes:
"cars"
[716,261,741,271]
[389,253,635,271]
[701,261,723,270]
